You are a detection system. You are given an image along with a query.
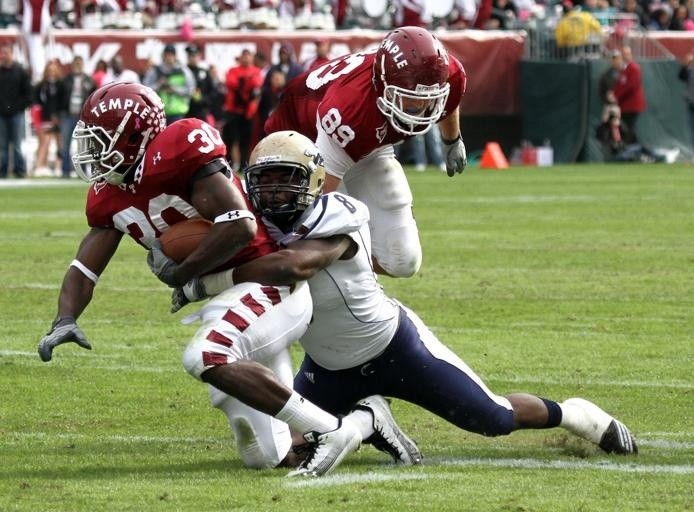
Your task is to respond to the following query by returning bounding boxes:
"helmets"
[69,81,166,183]
[373,25,451,137]
[244,130,328,216]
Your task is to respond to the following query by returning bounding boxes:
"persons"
[262,24,469,277]
[414,127,447,169]
[37,80,420,482]
[145,128,637,456]
[600,45,679,164]
[1,1,693,30]
[679,55,693,115]
[1,38,337,178]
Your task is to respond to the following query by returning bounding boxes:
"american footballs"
[160,218,213,264]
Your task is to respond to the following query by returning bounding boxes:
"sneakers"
[563,397,638,458]
[284,413,362,480]
[352,394,424,468]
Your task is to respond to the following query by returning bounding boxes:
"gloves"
[441,129,467,177]
[171,275,209,314]
[37,316,92,362]
[147,239,180,288]
